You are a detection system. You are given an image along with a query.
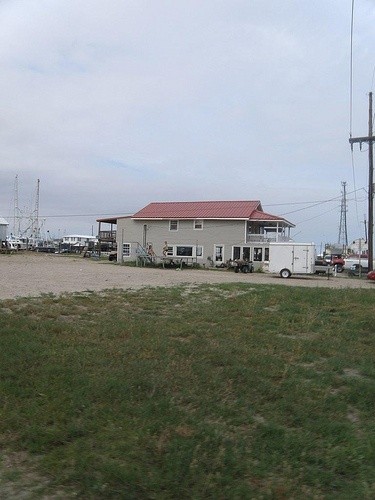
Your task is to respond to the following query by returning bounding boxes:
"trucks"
[330,255,344,273]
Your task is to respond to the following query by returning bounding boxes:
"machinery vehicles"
[229,260,254,273]
